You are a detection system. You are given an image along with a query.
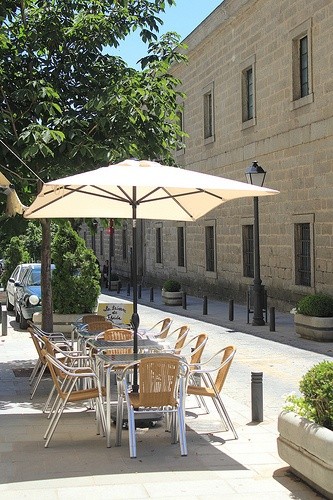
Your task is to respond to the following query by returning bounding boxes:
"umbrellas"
[23,158,281,385]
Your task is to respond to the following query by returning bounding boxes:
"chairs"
[26,313,236,457]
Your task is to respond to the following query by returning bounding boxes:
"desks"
[77,331,141,339]
[95,352,185,448]
[71,322,87,329]
[86,339,158,354]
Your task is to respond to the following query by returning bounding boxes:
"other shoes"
[105,286,108,288]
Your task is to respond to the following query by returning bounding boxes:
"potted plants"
[33,221,101,336]
[160,279,183,306]
[0,236,32,305]
[107,274,121,290]
[293,293,333,342]
[277,359,333,498]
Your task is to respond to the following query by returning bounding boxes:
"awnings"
[0,172,27,217]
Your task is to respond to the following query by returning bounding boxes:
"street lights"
[245,160,268,327]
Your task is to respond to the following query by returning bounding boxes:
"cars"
[6,263,56,329]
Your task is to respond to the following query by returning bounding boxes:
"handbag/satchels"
[104,273,107,278]
